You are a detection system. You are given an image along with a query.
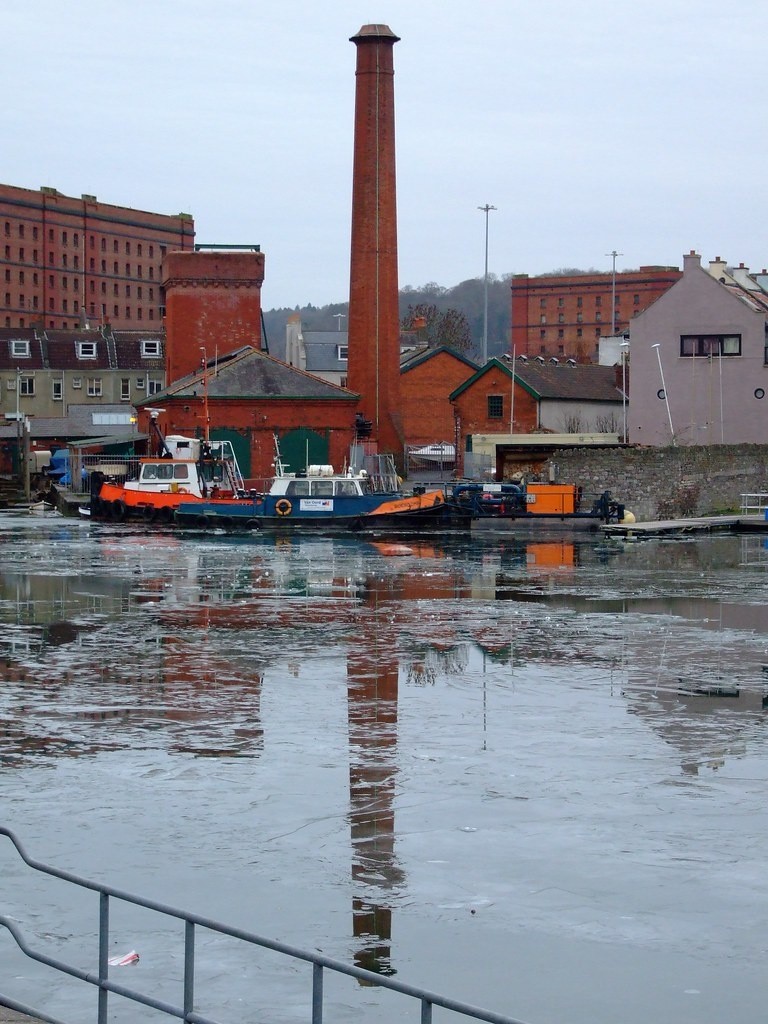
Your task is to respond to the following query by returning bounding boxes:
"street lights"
[15,367,24,482]
[620,342,630,444]
[605,251,624,336]
[333,313,346,331]
[477,203,498,366]
[650,344,676,446]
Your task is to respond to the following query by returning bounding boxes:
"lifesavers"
[94,495,174,523]
[245,519,261,529]
[195,514,210,529]
[220,516,236,529]
[178,487,187,493]
[275,499,292,515]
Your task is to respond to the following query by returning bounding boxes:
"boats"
[100,347,263,523]
[174,477,446,530]
[407,445,455,470]
[405,461,626,532]
